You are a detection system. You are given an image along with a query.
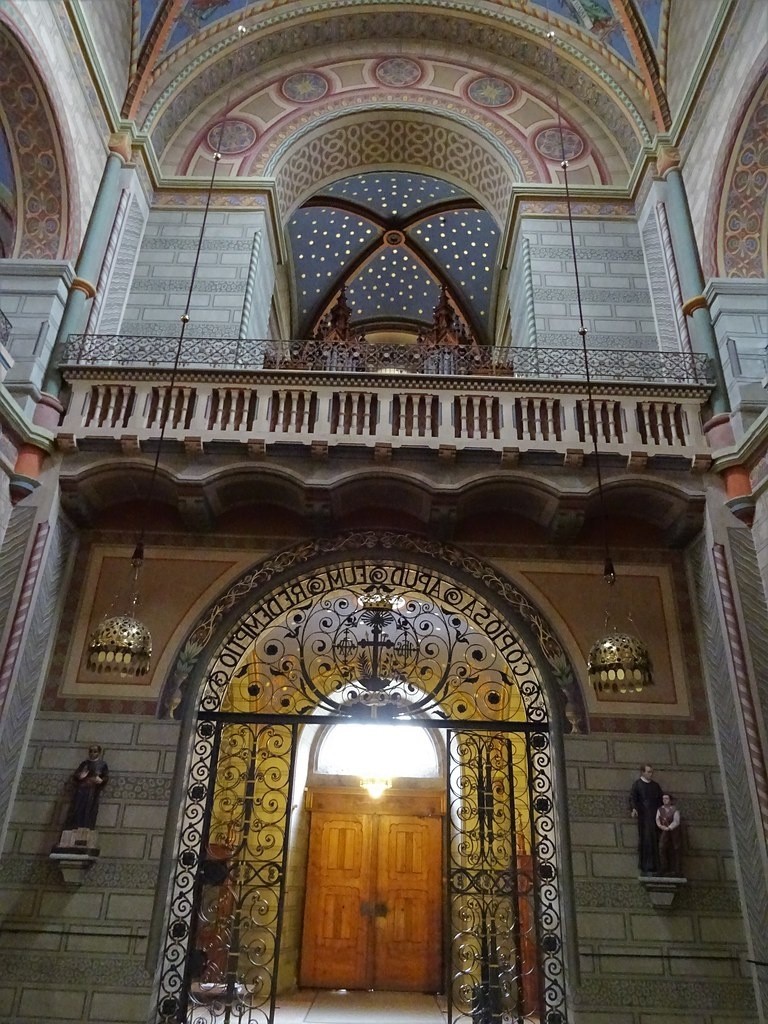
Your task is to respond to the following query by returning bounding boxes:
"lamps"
[536,0,651,695]
[84,0,253,676]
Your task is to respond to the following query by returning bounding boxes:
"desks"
[191,982,256,1024]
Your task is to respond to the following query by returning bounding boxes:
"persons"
[629,763,681,875]
[60,745,109,848]
